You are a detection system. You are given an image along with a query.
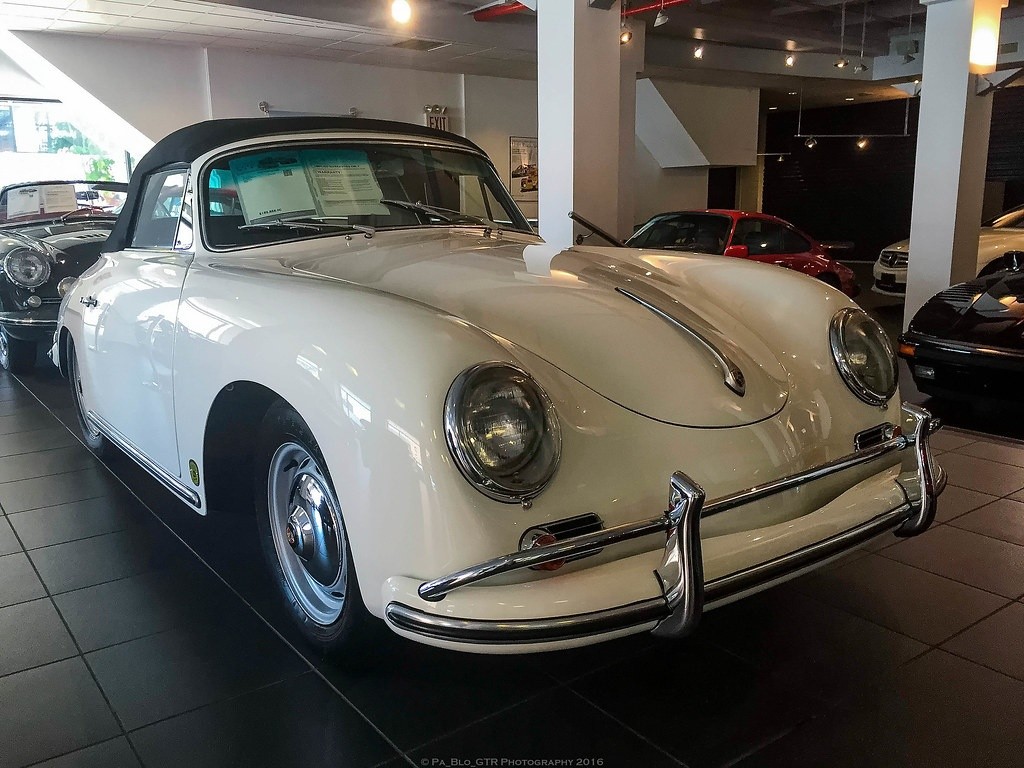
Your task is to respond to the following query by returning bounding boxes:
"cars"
[896,248,1024,418]
[873,202,1024,306]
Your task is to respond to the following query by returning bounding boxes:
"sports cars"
[47,116,950,672]
[0,179,174,378]
[623,209,856,302]
